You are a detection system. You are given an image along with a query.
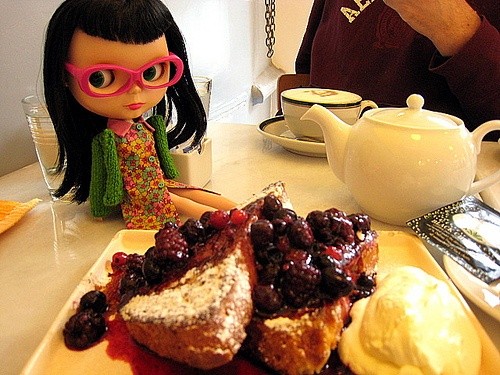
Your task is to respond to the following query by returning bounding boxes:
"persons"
[43,0,239,230]
[295,0,500,142]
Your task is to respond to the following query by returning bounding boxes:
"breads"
[118,199,257,367]
[252,225,378,375]
[226,180,294,219]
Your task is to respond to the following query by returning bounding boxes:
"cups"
[21,95,83,204]
[192,76,212,123]
[281,88,378,140]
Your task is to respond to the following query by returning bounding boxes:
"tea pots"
[299,94,500,225]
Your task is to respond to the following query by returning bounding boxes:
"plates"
[17,229,500,375]
[256,114,361,157]
